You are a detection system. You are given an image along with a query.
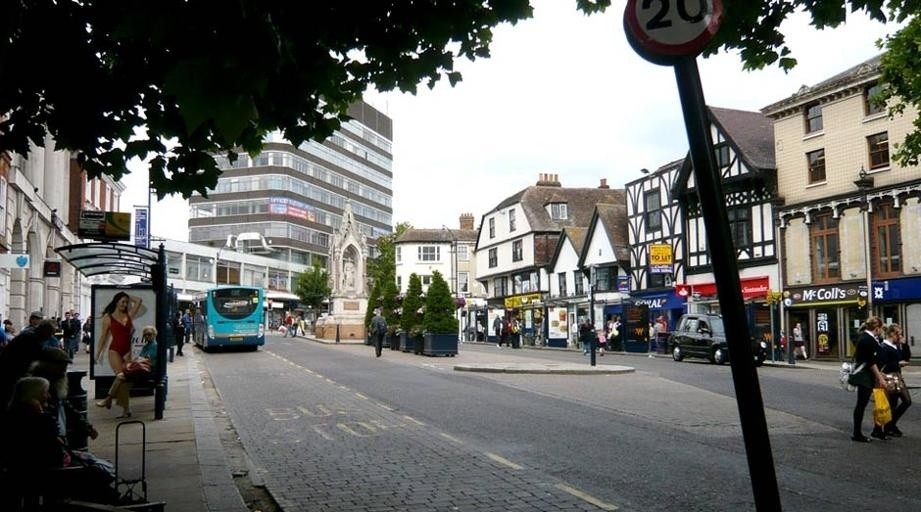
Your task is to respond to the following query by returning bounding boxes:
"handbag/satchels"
[874,372,906,394]
[121,357,152,381]
[839,362,858,392]
[376,321,388,336]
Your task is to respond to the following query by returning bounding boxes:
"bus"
[189,284,266,355]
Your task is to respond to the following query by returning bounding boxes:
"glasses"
[144,333,152,336]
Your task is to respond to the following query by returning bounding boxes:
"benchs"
[128,375,167,420]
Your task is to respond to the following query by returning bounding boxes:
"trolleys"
[109,418,150,506]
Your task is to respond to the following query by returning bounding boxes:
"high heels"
[96,399,112,409]
[115,410,132,419]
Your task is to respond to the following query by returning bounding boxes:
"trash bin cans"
[65,369,88,451]
[656,332,672,354]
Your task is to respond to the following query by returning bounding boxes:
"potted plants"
[363,272,457,358]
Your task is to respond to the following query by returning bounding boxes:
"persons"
[369,308,387,357]
[793,323,809,361]
[282,311,306,338]
[848,316,913,443]
[477,315,520,349]
[0,290,197,511]
[579,314,668,356]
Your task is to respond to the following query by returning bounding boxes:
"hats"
[31,311,43,318]
[38,348,73,365]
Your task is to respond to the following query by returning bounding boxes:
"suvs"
[664,311,769,371]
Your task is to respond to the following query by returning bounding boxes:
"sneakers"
[851,432,872,443]
[871,426,889,441]
[176,350,183,356]
[884,424,902,437]
